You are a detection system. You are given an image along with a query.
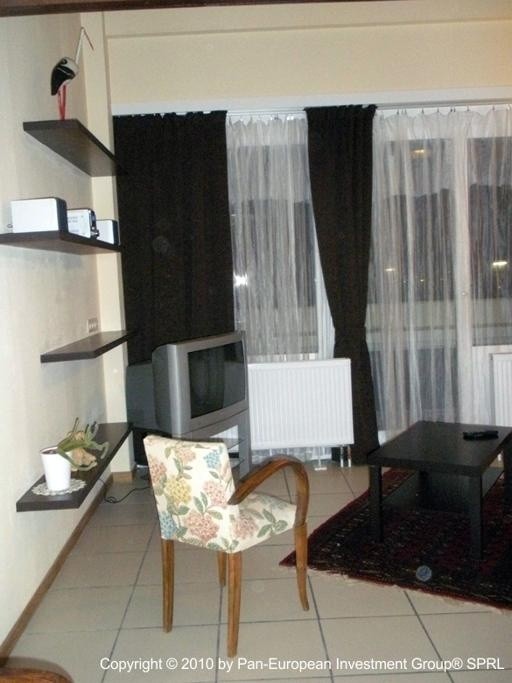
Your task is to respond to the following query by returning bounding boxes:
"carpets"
[278,467,511,613]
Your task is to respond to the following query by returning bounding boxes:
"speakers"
[63,207,99,241]
[96,220,119,245]
[11,197,69,233]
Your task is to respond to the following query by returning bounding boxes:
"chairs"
[141,433,311,662]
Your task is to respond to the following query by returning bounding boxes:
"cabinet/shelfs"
[131,408,252,480]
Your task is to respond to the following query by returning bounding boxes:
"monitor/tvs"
[125,330,250,434]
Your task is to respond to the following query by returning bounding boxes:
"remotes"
[463,430,498,437]
